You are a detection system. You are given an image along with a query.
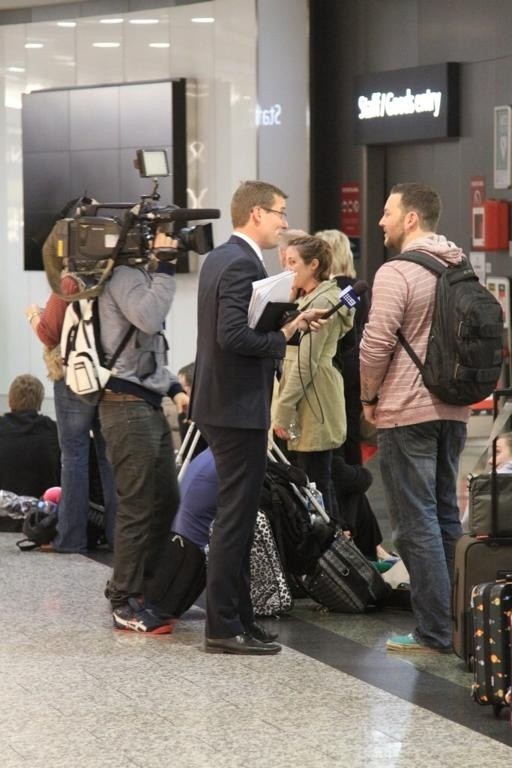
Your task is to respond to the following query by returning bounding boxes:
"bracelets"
[359,398,378,407]
[28,310,42,324]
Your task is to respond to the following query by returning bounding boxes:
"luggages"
[450,390,511,672]
[267,434,391,615]
[471,568,511,716]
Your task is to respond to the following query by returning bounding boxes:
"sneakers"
[112,604,173,634]
[385,634,440,654]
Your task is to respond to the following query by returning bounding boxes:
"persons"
[357,182,467,653]
[172,179,393,654]
[0,374,62,533]
[104,207,194,635]
[25,256,114,554]
[461,432,512,533]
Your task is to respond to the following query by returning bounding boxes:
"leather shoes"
[246,620,279,643]
[204,633,281,655]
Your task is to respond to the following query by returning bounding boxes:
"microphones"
[321,280,369,318]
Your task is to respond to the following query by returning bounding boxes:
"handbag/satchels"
[146,532,204,616]
[206,509,294,617]
[17,510,100,552]
[467,472,512,536]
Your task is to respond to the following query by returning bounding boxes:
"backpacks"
[59,263,151,407]
[382,251,506,406]
